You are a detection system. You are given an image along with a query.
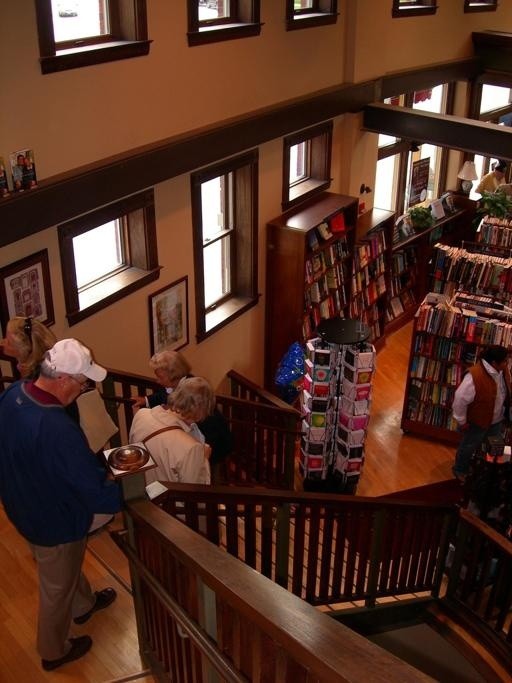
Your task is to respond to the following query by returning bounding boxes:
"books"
[308,347,336,479]
[355,230,390,268]
[475,217,512,256]
[353,277,388,314]
[308,213,350,251]
[418,306,512,345]
[414,334,479,362]
[348,350,376,474]
[361,301,387,322]
[304,286,348,337]
[390,289,419,318]
[305,263,351,314]
[306,237,353,283]
[410,378,453,401]
[433,243,511,297]
[412,358,464,385]
[391,270,424,294]
[404,399,462,428]
[335,350,360,473]
[370,323,384,341]
[392,245,423,276]
[352,254,385,292]
[298,338,314,481]
[451,287,512,322]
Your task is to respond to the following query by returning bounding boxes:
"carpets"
[334,478,470,561]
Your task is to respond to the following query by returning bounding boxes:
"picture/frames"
[148,274,189,357]
[0,248,55,339]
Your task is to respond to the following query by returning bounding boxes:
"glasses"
[23,317,33,347]
[68,375,89,392]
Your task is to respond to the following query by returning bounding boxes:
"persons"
[3,339,123,671]
[24,158,37,186]
[12,155,26,187]
[2,313,59,380]
[452,345,512,484]
[130,374,217,531]
[131,349,234,483]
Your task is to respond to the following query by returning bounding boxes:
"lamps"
[458,161,478,192]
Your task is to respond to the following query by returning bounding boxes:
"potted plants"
[406,204,434,229]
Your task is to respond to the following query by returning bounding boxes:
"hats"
[46,338,108,382]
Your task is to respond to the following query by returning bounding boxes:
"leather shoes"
[74,586,116,623]
[41,635,92,671]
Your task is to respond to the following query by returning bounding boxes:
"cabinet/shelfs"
[299,317,376,499]
[475,219,512,258]
[263,192,359,405]
[401,296,512,445]
[358,208,396,349]
[427,244,512,308]
[388,195,474,336]
